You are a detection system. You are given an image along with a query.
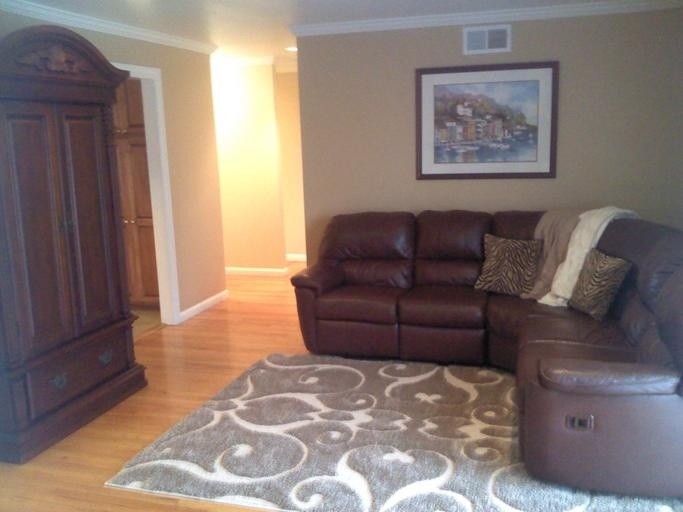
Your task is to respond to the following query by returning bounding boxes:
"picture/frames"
[415,61,559,179]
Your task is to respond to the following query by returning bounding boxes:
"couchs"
[291,210,683,499]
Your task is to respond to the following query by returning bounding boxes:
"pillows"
[474,233,543,297]
[567,247,632,322]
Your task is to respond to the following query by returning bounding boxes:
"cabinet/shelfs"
[0,26,148,466]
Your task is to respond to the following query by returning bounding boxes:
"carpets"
[105,353,683,512]
